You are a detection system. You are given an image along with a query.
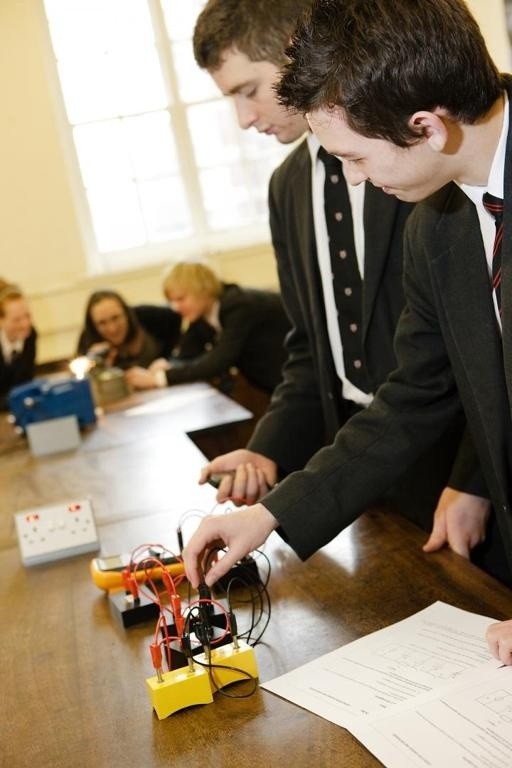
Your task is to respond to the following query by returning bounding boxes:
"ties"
[483,193,503,314]
[313,147,373,394]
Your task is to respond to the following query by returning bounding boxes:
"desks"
[1,362,511,768]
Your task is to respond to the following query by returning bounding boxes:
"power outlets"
[14,499,100,564]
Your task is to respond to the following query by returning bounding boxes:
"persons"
[73,285,205,378]
[191,4,490,563]
[0,280,39,415]
[128,256,285,400]
[179,2,512,675]
